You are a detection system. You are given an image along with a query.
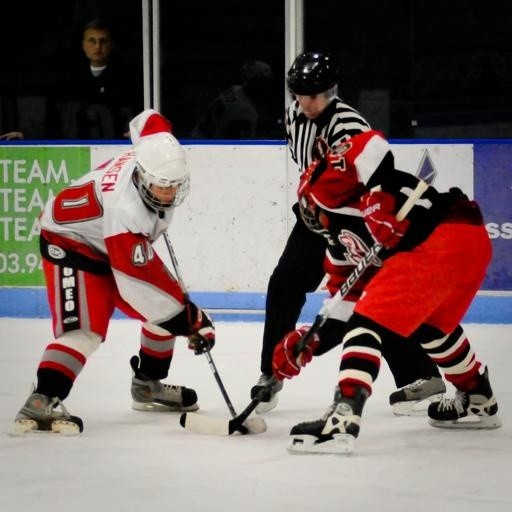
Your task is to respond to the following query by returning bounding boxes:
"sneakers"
[390,374,446,403]
[250,373,283,402]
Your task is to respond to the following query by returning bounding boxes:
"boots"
[428,367,498,421]
[17,392,83,433]
[291,387,369,438]
[130,354,197,406]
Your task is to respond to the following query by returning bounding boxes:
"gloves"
[360,191,411,251]
[272,326,321,381]
[183,307,215,353]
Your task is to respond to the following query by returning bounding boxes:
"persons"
[0,19,143,141]
[284,130,502,455]
[7,110,216,437]
[188,72,284,140]
[251,52,447,415]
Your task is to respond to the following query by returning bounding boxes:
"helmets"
[286,52,340,96]
[297,160,330,231]
[135,139,191,212]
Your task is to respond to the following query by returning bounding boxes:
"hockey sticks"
[162,231,267,435]
[180,178,429,434]
